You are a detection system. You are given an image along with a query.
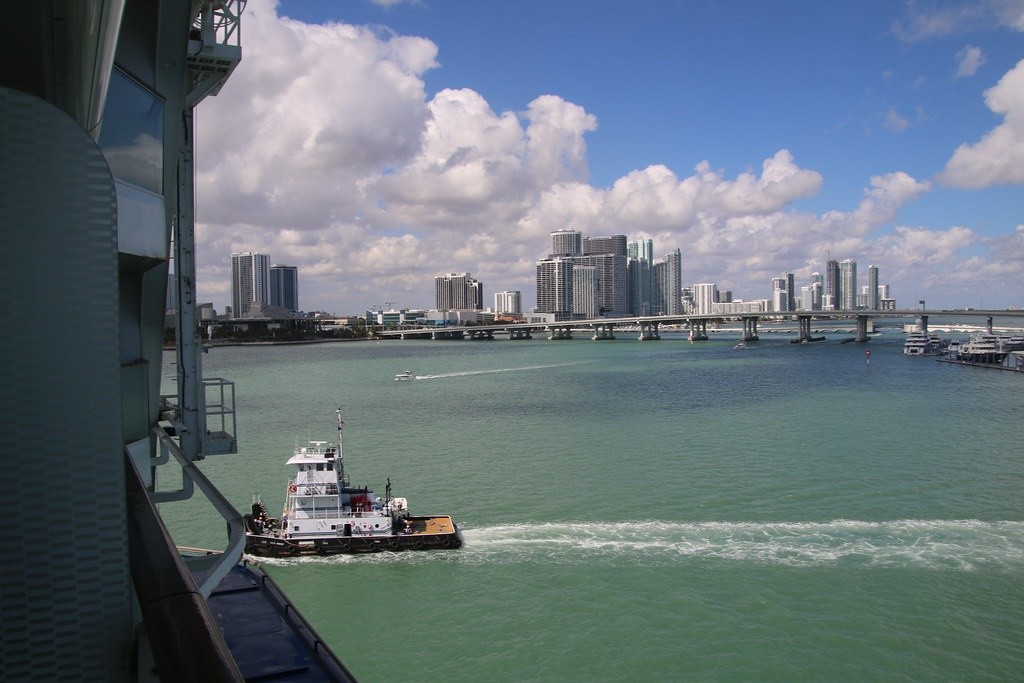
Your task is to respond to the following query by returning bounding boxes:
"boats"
[394,370,417,381]
[903,334,941,357]
[732,343,757,351]
[225,407,462,557]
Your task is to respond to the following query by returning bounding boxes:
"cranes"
[370,302,398,311]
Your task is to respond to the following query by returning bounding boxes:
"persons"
[258,517,264,531]
